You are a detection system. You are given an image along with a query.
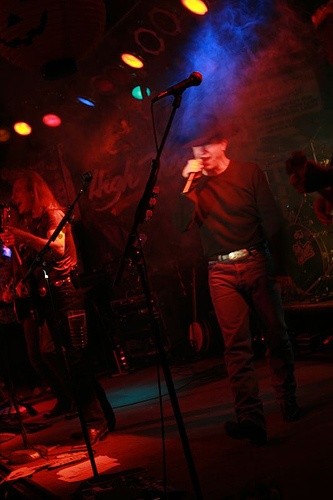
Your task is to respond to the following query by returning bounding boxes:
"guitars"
[4,205,54,324]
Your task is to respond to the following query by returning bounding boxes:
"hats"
[184,114,222,146]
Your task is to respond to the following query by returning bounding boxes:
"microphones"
[152,71,202,102]
[182,159,204,194]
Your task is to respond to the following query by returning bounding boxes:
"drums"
[280,220,333,296]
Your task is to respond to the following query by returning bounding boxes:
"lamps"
[0,0,222,145]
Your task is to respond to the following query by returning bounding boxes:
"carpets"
[104,377,190,408]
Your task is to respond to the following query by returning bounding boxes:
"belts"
[208,247,258,264]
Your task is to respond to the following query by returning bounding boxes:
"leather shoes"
[281,396,299,422]
[31,408,79,427]
[226,418,266,446]
[70,415,117,447]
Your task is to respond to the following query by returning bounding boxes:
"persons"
[285,150,333,226]
[0,170,95,418]
[172,113,298,445]
